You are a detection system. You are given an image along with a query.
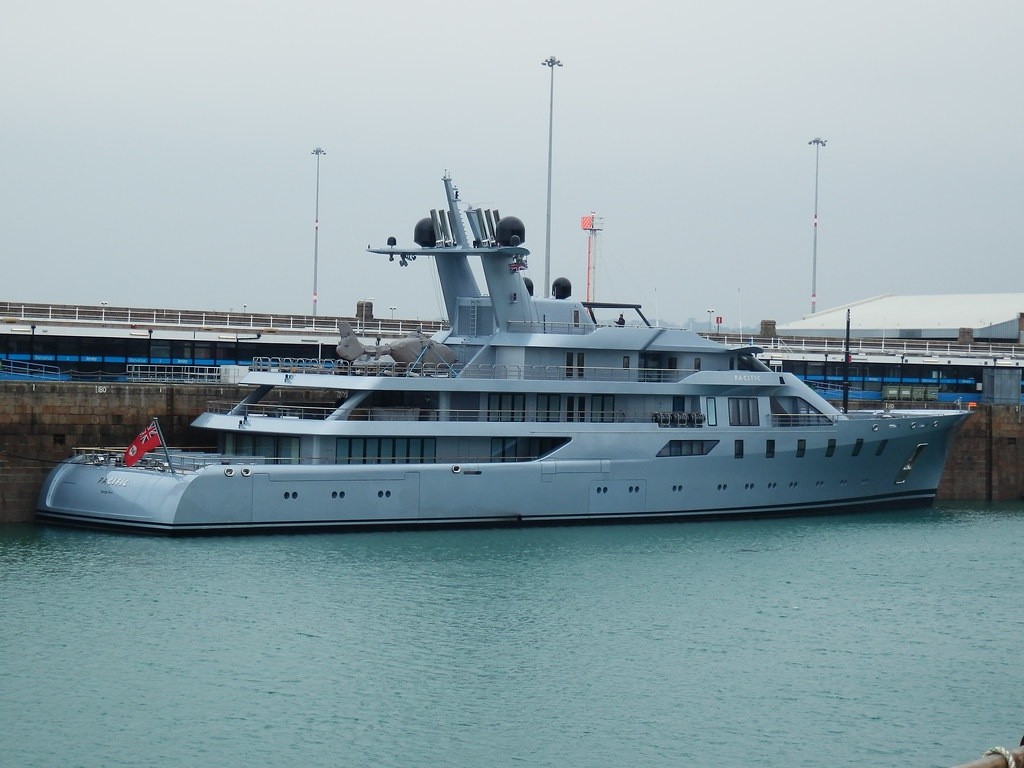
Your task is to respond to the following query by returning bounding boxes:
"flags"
[509,262,527,273]
[124,422,161,467]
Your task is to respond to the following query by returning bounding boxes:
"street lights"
[310,147,329,314]
[808,139,834,315]
[540,56,567,298]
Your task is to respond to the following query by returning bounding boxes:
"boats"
[37,168,978,535]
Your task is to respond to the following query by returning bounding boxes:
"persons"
[614,314,625,327]
